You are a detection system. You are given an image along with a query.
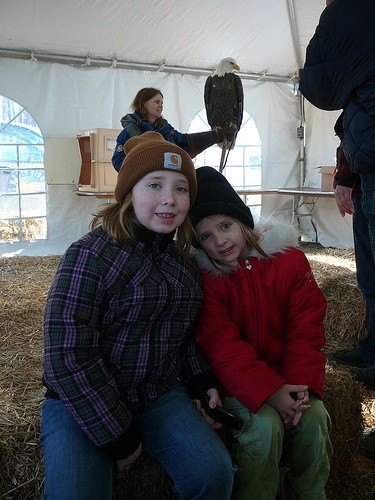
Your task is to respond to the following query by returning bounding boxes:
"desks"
[74,191,117,207]
[240,187,338,244]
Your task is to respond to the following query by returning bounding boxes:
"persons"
[111,88,222,173]
[299,0,374,394]
[188,167,333,500]
[40,131,237,500]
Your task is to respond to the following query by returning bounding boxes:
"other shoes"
[360,429,375,460]
[333,350,375,367]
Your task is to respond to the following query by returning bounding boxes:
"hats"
[189,166,254,250]
[115,131,198,213]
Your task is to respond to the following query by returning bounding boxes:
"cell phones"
[199,391,242,430]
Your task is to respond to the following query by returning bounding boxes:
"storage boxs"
[314,165,338,192]
